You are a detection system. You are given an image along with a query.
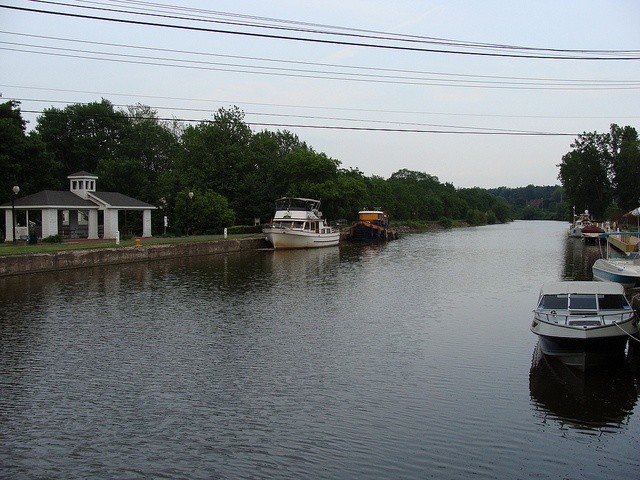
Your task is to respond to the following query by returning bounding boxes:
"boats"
[593,232,635,281]
[581,225,605,238]
[350,209,401,244]
[264,196,342,248]
[530,280,635,354]
[569,206,593,237]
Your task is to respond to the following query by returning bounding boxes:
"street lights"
[185,191,194,237]
[10,184,20,243]
